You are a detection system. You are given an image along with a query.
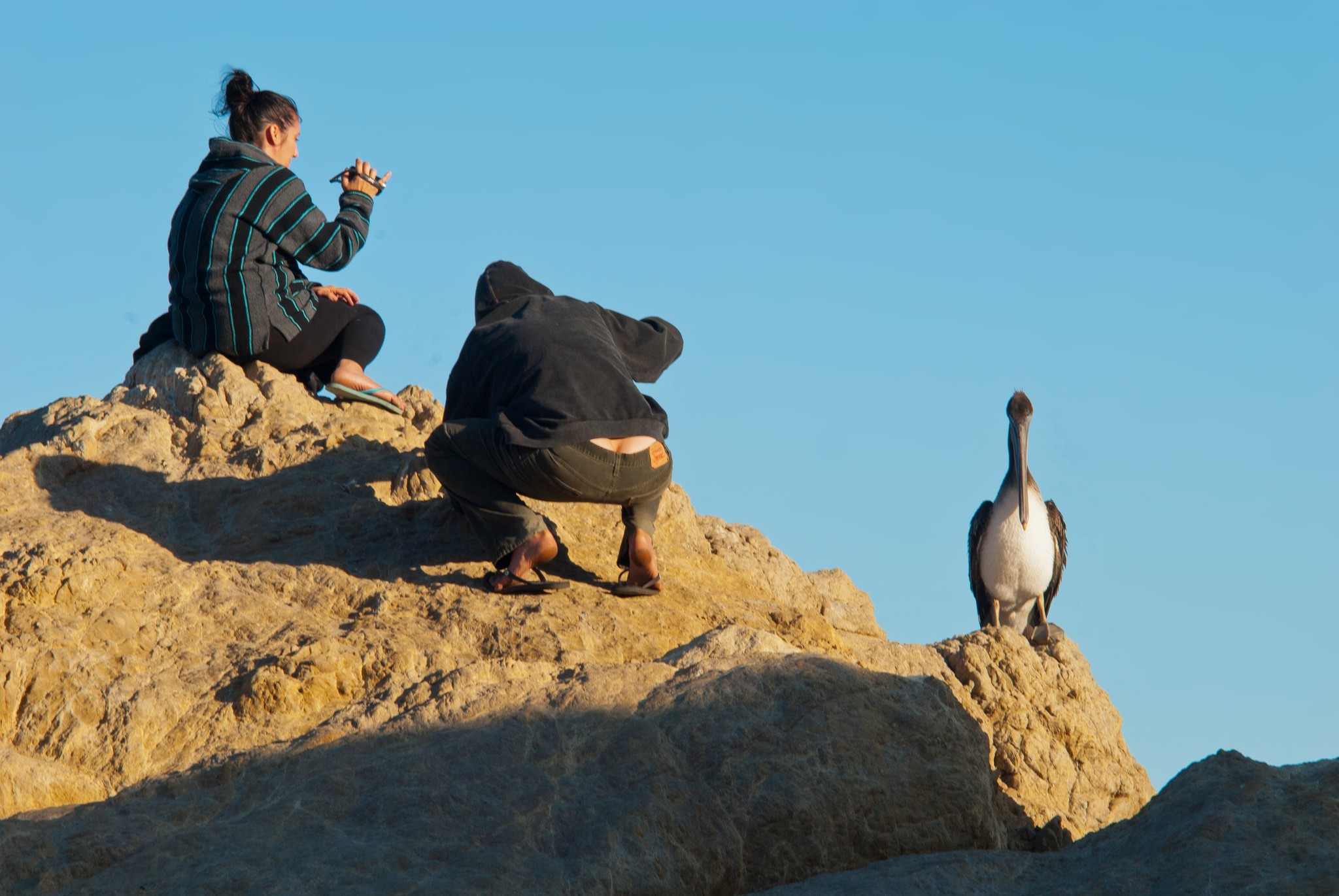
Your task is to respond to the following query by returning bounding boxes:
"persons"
[167,69,406,414]
[424,259,684,594]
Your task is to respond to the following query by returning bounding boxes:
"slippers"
[490,566,570,596]
[611,567,661,597]
[326,380,404,415]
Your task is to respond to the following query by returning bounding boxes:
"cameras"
[347,166,386,196]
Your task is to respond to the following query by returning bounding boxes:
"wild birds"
[967,398,1073,639]
